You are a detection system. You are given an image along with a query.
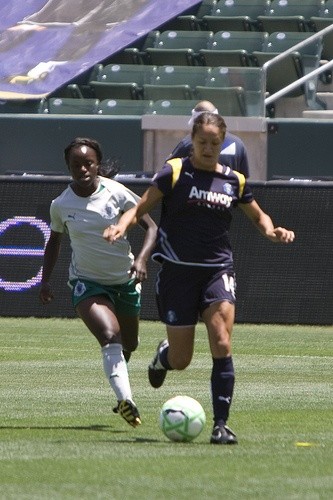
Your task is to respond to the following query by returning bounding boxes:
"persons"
[164,101,249,180]
[40,137,158,427]
[103,112,295,444]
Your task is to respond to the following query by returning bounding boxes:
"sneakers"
[210,419,237,444]
[150,339,170,388]
[112,399,141,428]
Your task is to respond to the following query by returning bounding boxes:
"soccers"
[158,395,207,443]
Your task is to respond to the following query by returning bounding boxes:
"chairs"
[0,0,333,183]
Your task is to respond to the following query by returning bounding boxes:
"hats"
[188,108,218,125]
